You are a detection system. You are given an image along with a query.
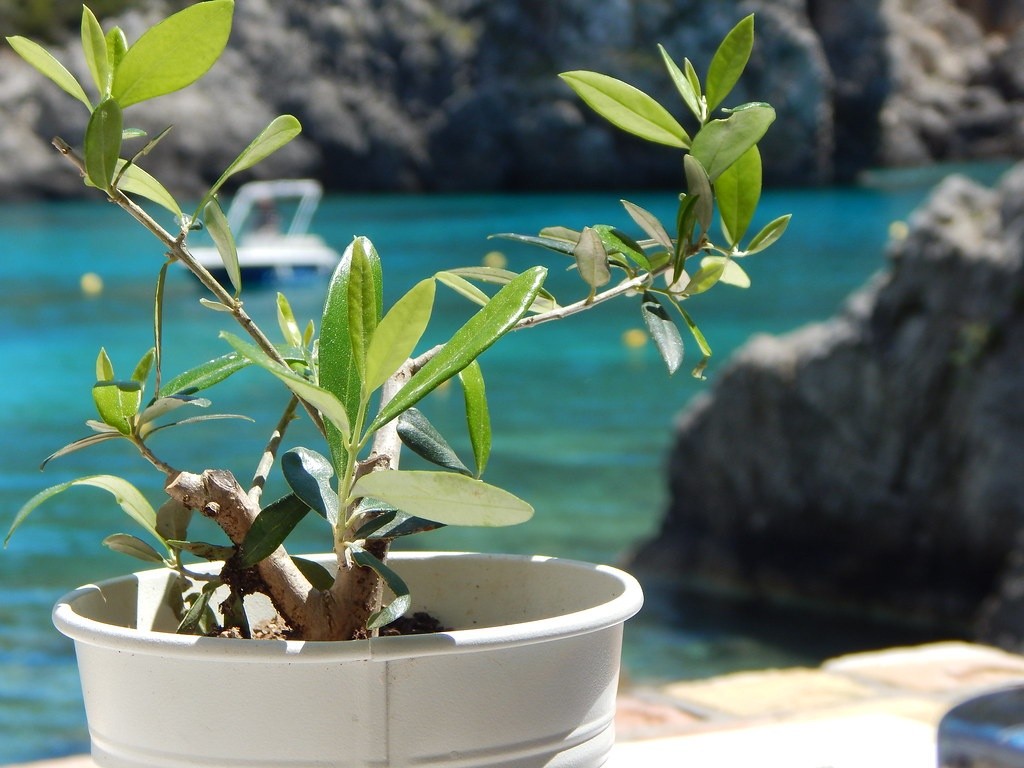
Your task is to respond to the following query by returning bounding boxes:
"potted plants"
[0,2,791,767]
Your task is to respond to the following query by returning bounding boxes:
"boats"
[171,178,351,293]
